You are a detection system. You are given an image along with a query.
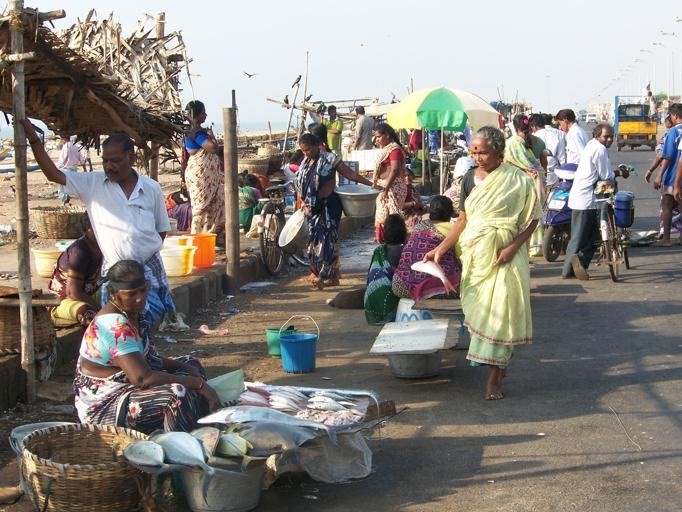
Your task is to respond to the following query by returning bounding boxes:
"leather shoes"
[561,273,573,279]
[571,254,588,280]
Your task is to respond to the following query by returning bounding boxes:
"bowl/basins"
[333,181,381,220]
[31,247,64,277]
[278,208,312,256]
[386,352,444,379]
[160,245,197,277]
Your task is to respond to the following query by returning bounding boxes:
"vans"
[586,113,598,125]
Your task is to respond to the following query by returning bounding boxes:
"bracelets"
[238,176,268,234]
[371,181,377,191]
[198,374,204,391]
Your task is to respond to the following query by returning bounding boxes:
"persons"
[44,210,105,327]
[644,109,676,239]
[648,91,655,116]
[10,115,174,338]
[544,115,568,164]
[408,129,423,156]
[321,105,343,186]
[158,309,191,332]
[373,124,408,244]
[555,109,590,182]
[302,123,342,281]
[72,259,222,434]
[165,186,195,231]
[673,138,682,210]
[239,169,272,200]
[409,125,545,401]
[278,133,387,291]
[560,123,630,282]
[288,148,305,175]
[392,195,463,300]
[183,101,220,252]
[528,113,566,193]
[647,102,682,248]
[502,113,547,266]
[353,105,372,150]
[428,131,440,152]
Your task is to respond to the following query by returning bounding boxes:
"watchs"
[618,170,624,176]
[28,138,42,145]
[647,169,652,175]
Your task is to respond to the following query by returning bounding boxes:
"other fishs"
[190,425,241,483]
[123,439,198,479]
[196,405,342,449]
[215,422,268,472]
[235,385,361,418]
[143,428,249,508]
[410,260,459,296]
[225,421,320,469]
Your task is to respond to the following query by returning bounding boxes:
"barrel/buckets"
[277,314,319,374]
[182,233,217,270]
[8,422,77,494]
[265,328,297,358]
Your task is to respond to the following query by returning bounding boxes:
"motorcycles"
[541,149,619,262]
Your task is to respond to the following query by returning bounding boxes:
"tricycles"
[614,96,658,151]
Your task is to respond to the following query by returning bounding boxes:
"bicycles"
[257,180,312,277]
[594,181,631,283]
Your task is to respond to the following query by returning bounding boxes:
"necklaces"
[108,298,129,322]
